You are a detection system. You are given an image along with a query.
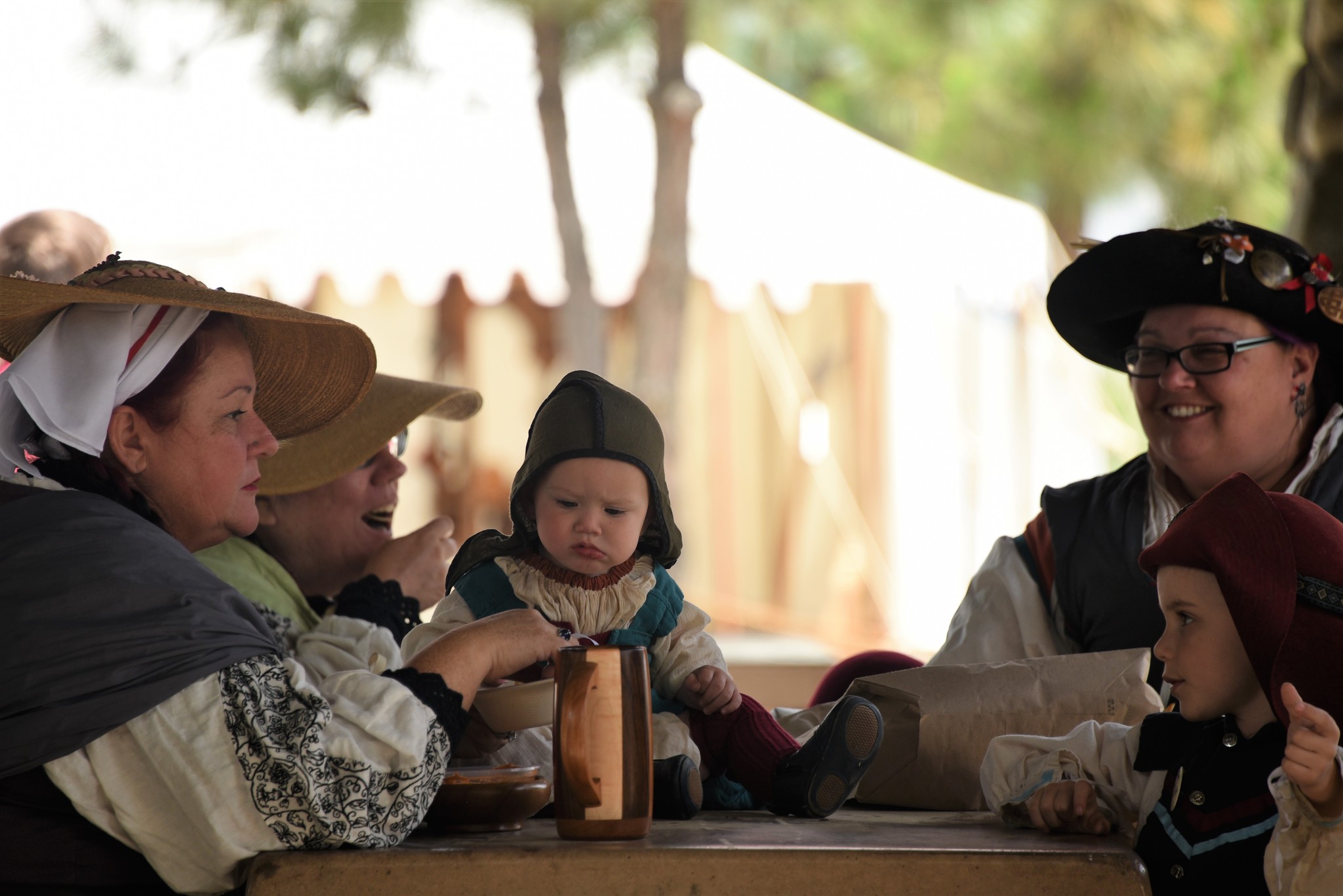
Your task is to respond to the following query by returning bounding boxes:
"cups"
[554,645,653,840]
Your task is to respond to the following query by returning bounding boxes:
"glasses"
[1122,336,1279,380]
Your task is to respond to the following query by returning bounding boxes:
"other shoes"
[653,755,703,819]
[764,693,884,822]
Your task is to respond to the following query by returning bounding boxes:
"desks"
[248,806,1151,895]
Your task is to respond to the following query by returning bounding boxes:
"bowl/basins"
[471,678,557,732]
[442,765,541,783]
[425,776,552,833]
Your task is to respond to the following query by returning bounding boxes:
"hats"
[444,369,684,597]
[255,371,483,496]
[1136,471,1343,739]
[1046,219,1343,406]
[0,251,377,445]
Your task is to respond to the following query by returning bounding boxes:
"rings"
[508,732,516,742]
[557,627,571,640]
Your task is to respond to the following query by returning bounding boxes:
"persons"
[400,371,883,822]
[0,261,582,896]
[924,219,1343,713]
[977,470,1343,896]
[0,209,112,473]
